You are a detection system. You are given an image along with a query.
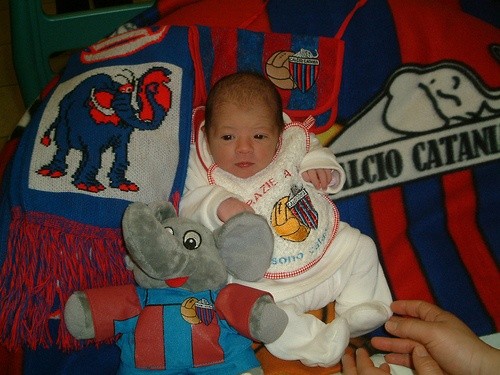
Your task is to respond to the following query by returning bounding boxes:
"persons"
[341,299,500,375]
[178,72,395,368]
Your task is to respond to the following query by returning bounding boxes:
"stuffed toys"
[65,201,289,375]
